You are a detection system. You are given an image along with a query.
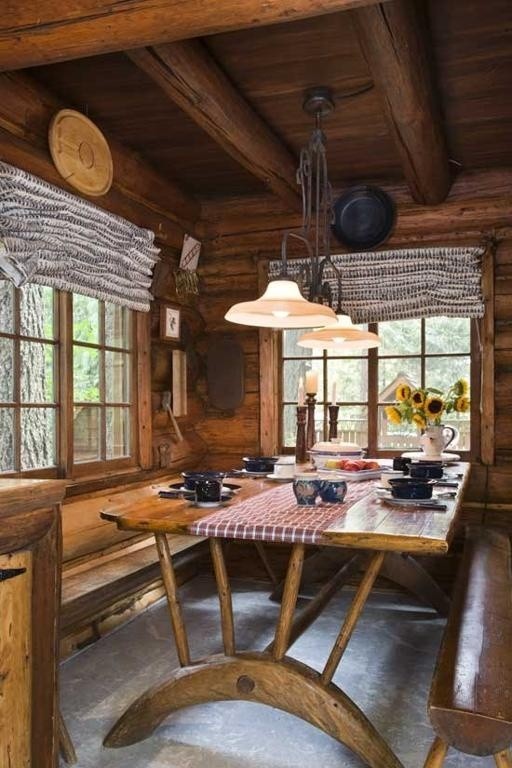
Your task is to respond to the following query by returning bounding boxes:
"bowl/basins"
[388,476,440,497]
[406,461,447,480]
[180,471,227,490]
[239,454,280,471]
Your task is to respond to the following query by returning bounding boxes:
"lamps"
[224,83,385,353]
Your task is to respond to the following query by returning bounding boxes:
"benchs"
[423,529,510,768]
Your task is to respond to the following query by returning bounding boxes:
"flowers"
[385,380,471,427]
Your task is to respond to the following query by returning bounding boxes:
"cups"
[195,480,221,502]
[294,473,347,506]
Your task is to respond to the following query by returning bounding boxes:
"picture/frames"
[159,303,183,348]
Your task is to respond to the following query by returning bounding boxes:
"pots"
[308,438,365,470]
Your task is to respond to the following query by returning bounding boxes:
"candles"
[296,368,338,405]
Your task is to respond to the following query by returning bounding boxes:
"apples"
[324,458,380,471]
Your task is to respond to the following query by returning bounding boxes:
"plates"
[168,482,243,508]
[235,469,294,483]
[381,493,440,507]
